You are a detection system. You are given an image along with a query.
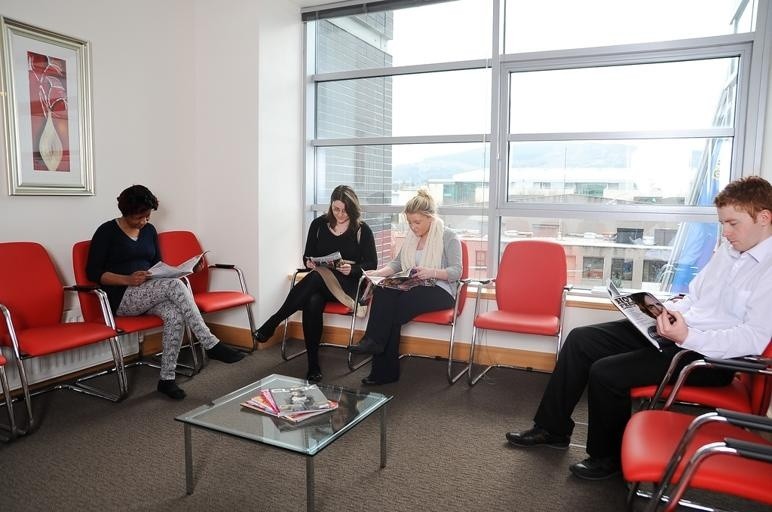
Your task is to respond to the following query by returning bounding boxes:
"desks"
[172,372,400,512]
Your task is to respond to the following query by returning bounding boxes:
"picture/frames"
[1,11,100,199]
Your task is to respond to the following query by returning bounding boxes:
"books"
[360,267,425,292]
[240,384,338,423]
[305,251,345,271]
[607,280,690,352]
[146,250,210,279]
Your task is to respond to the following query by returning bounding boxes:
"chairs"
[366,238,485,385]
[615,355,772,512]
[621,324,771,512]
[67,234,200,402]
[1,240,127,439]
[0,354,17,446]
[465,238,572,390]
[278,254,380,375]
[152,227,260,373]
[663,439,772,512]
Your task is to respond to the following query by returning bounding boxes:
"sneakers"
[206,341,247,364]
[158,379,187,401]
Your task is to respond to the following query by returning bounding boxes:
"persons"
[505,176,772,482]
[252,184,378,381]
[316,384,369,434]
[348,187,463,385]
[631,292,674,349]
[85,185,245,400]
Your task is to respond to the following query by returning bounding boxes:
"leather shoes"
[252,323,275,342]
[503,427,572,450]
[566,457,623,482]
[307,368,323,382]
[362,369,403,385]
[345,338,386,356]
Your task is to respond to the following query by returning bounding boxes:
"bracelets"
[434,269,436,279]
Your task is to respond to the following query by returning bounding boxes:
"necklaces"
[339,231,343,235]
